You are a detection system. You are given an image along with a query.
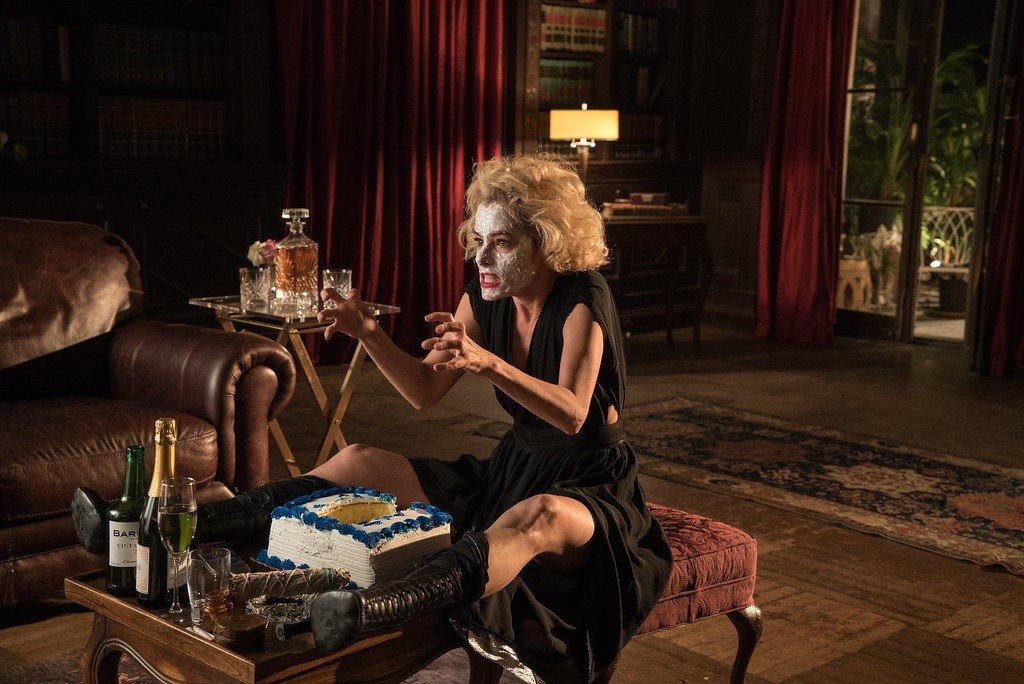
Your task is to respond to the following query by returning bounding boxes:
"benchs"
[917,268,971,321]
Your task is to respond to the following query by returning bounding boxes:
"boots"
[283,526,490,653]
[71,475,338,555]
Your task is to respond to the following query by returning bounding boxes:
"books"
[538,3,670,159]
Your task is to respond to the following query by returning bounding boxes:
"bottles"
[135,417,183,606]
[106,444,145,597]
[273,208,319,317]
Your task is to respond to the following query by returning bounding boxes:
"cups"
[322,269,352,309]
[239,267,271,313]
[185,548,231,624]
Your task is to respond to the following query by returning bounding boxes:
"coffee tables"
[63,566,505,684]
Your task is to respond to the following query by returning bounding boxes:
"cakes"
[257,486,453,587]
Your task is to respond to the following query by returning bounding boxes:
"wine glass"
[157,477,197,622]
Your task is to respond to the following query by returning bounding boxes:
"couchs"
[0,217,297,615]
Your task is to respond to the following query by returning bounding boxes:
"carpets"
[476,394,1023,578]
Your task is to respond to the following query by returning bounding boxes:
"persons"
[71,157,674,684]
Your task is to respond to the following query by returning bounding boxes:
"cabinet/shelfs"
[0,0,279,325]
[507,0,699,332]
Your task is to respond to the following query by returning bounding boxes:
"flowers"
[247,239,277,267]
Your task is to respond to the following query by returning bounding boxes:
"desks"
[602,213,716,356]
[188,297,402,477]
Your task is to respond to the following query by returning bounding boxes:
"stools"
[607,501,764,684]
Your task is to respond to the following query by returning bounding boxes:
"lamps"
[548,110,620,182]
